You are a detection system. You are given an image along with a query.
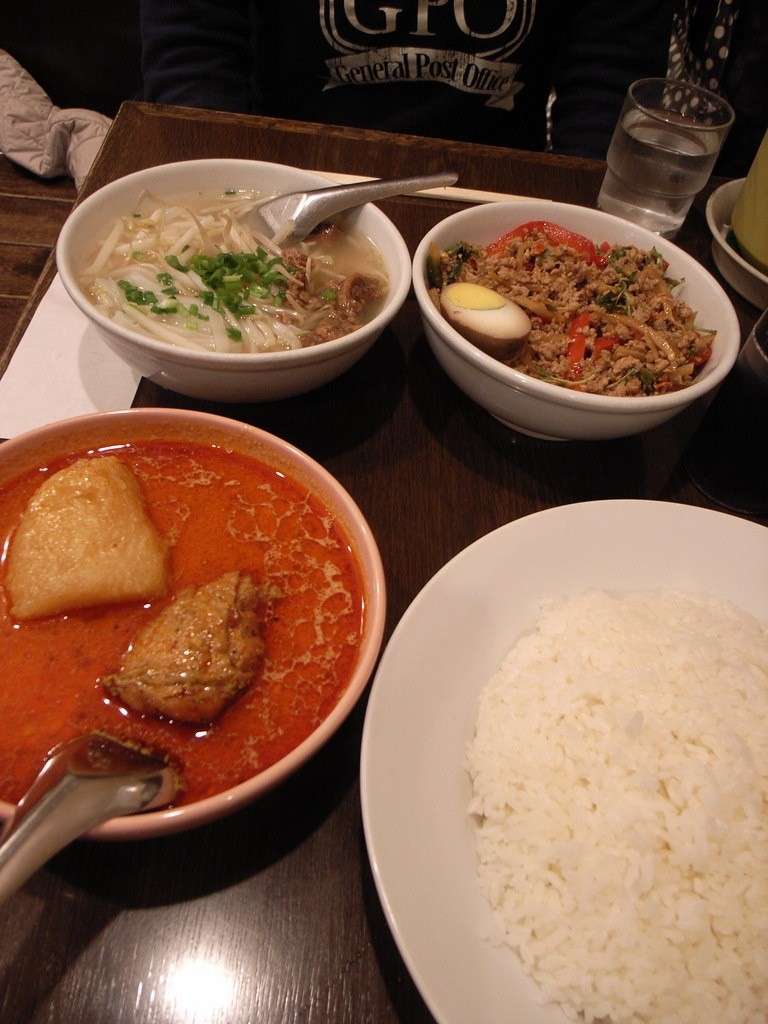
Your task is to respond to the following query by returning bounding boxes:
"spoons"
[236,171,458,250]
[0,738,180,903]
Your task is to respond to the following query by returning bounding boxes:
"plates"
[361,497,768,1024]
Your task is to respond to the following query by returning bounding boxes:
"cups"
[681,306,768,517]
[597,78,735,241]
[732,128,768,277]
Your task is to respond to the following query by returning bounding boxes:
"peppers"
[563,310,597,390]
[483,220,606,268]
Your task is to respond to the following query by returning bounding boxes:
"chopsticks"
[300,168,554,204]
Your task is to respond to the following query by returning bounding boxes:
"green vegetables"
[426,241,718,392]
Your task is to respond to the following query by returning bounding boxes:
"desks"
[0,99,768,1024]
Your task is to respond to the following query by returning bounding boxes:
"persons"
[140,0,646,161]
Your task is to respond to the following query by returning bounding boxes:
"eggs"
[440,281,532,355]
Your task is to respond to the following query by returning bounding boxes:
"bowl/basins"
[55,159,412,403]
[2,408,387,841]
[706,175,768,311]
[414,200,741,443]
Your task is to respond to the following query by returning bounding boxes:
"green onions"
[114,250,338,339]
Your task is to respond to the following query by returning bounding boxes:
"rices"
[465,588,768,1024]
